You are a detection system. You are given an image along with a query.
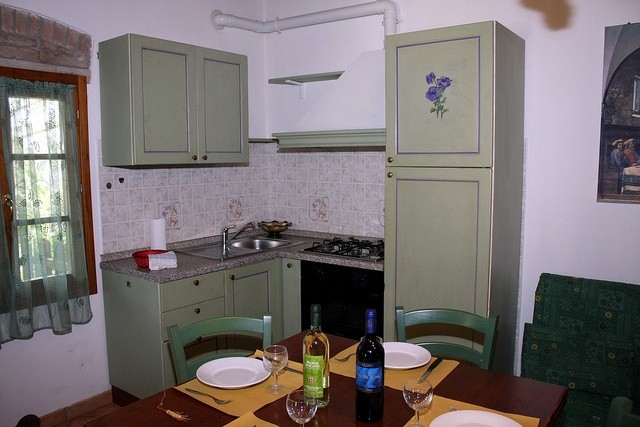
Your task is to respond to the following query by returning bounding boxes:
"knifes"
[255,357,304,377]
[418,356,443,385]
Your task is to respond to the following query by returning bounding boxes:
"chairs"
[396,305,499,372]
[168,315,272,389]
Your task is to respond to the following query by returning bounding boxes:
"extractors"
[272,48,385,150]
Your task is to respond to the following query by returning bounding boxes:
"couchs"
[520,271,639,426]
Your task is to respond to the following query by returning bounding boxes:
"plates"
[258,220,291,232]
[383,342,432,370]
[430,410,523,427]
[196,357,271,390]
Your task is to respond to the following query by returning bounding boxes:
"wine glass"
[263,345,288,395]
[403,379,433,427]
[287,390,317,427]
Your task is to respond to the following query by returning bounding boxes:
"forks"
[335,353,356,363]
[184,387,232,405]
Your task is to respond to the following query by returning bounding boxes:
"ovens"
[300,259,384,344]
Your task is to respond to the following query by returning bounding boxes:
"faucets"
[222,221,255,245]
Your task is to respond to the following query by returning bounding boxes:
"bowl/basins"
[131,249,173,269]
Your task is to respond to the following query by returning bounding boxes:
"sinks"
[231,239,293,250]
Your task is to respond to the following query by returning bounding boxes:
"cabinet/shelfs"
[98,35,250,166]
[100,263,226,409]
[385,20,526,377]
[227,257,284,360]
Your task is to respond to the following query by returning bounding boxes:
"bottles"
[303,304,330,409]
[355,309,384,423]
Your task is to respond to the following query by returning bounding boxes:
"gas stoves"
[299,236,384,263]
